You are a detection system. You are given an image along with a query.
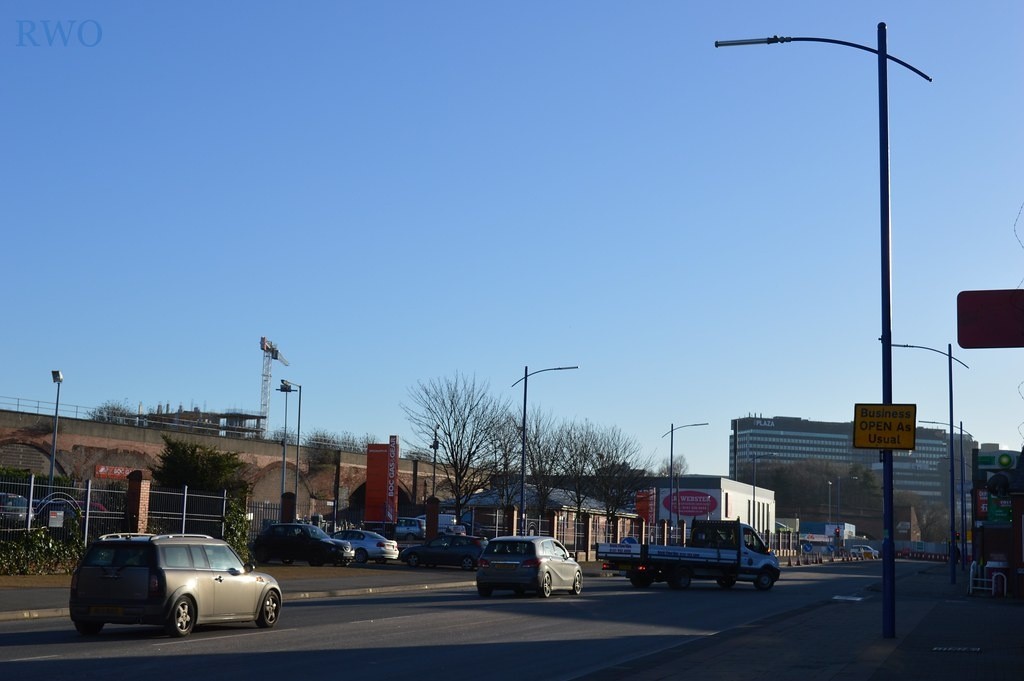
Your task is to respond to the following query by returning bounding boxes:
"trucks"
[596,516,781,592]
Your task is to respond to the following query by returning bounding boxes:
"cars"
[251,523,355,568]
[475,536,584,598]
[69,533,283,637]
[851,545,878,558]
[397,513,466,540]
[399,535,489,571]
[1,492,108,534]
[327,530,399,566]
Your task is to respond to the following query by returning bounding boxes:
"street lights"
[512,365,580,536]
[714,23,933,643]
[827,477,858,525]
[920,420,974,586]
[662,423,710,545]
[747,453,776,530]
[50,370,63,485]
[891,343,969,595]
[281,379,302,523]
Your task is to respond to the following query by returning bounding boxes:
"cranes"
[257,336,289,442]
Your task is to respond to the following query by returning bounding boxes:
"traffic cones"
[787,551,866,566]
